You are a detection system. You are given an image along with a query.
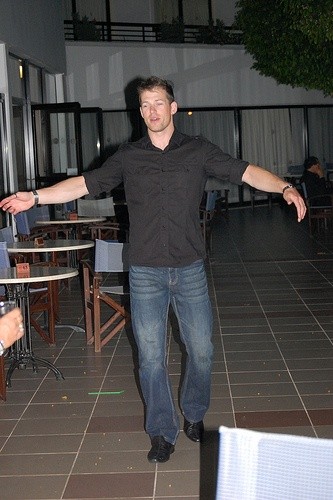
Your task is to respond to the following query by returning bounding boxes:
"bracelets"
[283,184,295,193]
[32,190,39,205]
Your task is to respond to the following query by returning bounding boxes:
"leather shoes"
[148,436,175,463]
[184,417,204,442]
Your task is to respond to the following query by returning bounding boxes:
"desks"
[8,239,94,332]
[0,265,80,388]
[36,216,107,269]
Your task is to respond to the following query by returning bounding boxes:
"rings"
[15,193,17,198]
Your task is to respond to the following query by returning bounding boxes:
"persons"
[0,77,307,462]
[302,155,333,206]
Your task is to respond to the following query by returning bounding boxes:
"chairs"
[199,189,230,258]
[249,177,333,236]
[216,426,333,500]
[0,197,131,353]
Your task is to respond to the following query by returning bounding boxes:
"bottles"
[15,251,25,264]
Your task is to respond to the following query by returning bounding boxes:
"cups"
[0,301,16,317]
